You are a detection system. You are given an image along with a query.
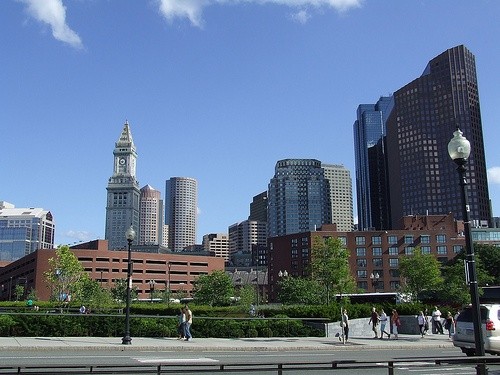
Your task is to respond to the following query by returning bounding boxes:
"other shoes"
[388,334,390,338]
[177,338,181,340]
[373,336,377,339]
[188,337,192,341]
[379,337,383,339]
[184,338,188,341]
[392,338,398,340]
[180,338,185,339]
[339,337,341,341]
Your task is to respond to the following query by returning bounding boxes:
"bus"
[332,292,412,304]
[118,297,243,304]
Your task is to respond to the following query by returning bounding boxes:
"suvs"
[451,303,500,356]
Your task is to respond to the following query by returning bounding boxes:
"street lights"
[278,270,288,306]
[150,279,155,303]
[55,265,62,314]
[121,225,136,343]
[448,128,488,374]
[370,273,380,293]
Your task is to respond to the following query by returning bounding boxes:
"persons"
[27,298,34,310]
[175,305,192,341]
[391,309,399,340]
[454,312,460,327]
[444,311,454,338]
[378,308,390,339]
[369,306,379,339]
[433,306,445,334]
[339,309,350,342]
[78,305,92,314]
[417,308,428,338]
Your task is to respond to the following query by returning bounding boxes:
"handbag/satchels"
[340,321,346,328]
